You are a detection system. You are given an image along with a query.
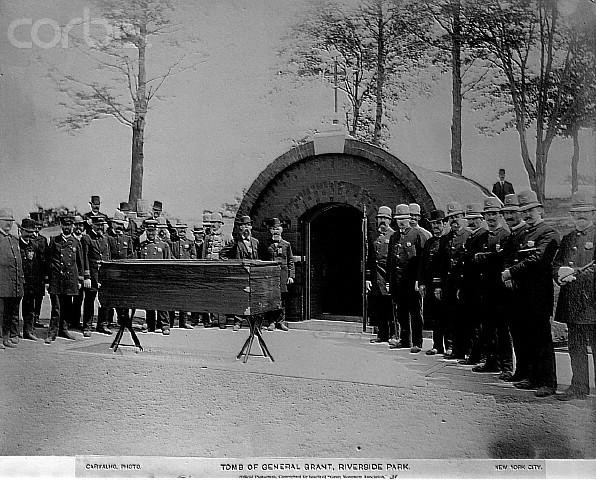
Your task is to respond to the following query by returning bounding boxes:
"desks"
[97,258,283,362]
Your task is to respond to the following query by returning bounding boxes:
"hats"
[376,203,420,220]
[426,191,543,223]
[143,201,188,231]
[191,213,223,233]
[568,191,596,213]
[60,197,107,226]
[271,218,282,227]
[238,215,252,224]
[0,209,44,232]
[111,202,131,225]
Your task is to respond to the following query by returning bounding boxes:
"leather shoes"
[498,370,555,396]
[0,333,37,349]
[267,324,275,331]
[203,319,225,329]
[370,337,411,349]
[162,329,170,334]
[143,326,154,333]
[45,326,90,343]
[179,322,194,329]
[234,323,240,330]
[426,348,502,372]
[557,388,586,401]
[96,324,112,334]
[276,323,289,331]
[411,346,420,353]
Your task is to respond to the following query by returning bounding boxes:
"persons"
[364,189,561,398]
[550,190,596,401]
[490,168,517,204]
[0,193,297,348]
